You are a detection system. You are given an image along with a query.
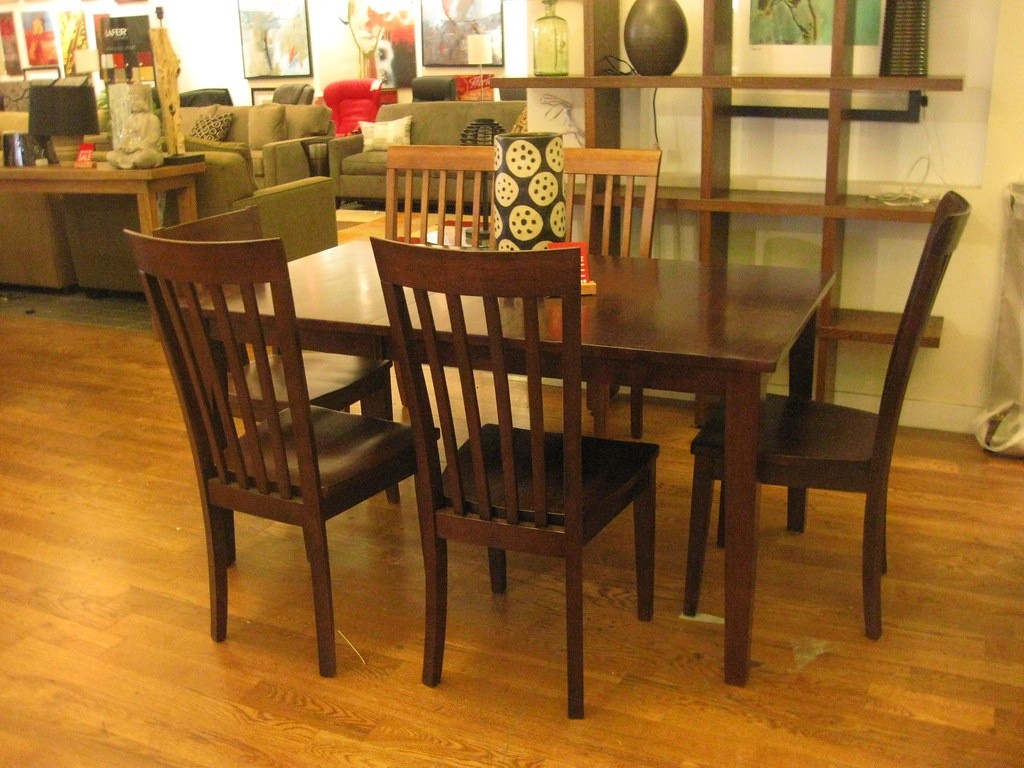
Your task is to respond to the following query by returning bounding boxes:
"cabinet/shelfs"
[489,0,963,394]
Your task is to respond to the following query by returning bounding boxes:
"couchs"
[318,80,397,137]
[327,102,532,215]
[61,150,339,299]
[178,104,337,190]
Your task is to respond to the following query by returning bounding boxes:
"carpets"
[335,209,386,231]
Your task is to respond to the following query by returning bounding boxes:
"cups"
[3,133,35,166]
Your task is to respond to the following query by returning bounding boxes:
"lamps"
[30,84,101,168]
[493,133,567,253]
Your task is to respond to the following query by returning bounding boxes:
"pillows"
[358,114,413,153]
[247,106,288,150]
[285,104,332,139]
[189,112,235,143]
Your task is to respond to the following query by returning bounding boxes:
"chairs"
[273,84,314,108]
[179,88,234,108]
[119,145,972,720]
[411,76,457,102]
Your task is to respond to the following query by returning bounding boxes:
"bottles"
[533,0,570,77]
[623,0,687,77]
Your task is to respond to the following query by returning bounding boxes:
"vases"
[534,0,571,76]
[624,0,689,77]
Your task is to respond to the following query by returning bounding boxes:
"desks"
[173,237,838,689]
[0,158,206,240]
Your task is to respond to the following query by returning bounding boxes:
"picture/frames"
[714,0,924,124]
[251,87,277,106]
[421,0,504,67]
[237,0,313,80]
